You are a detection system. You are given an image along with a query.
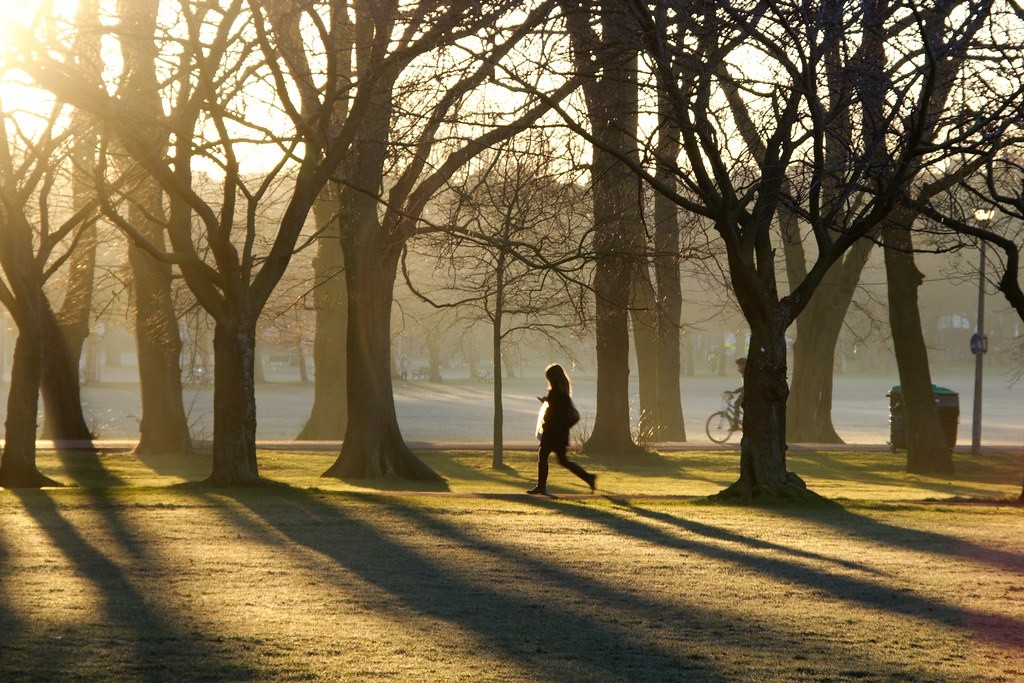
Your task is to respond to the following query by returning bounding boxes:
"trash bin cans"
[886,384,959,454]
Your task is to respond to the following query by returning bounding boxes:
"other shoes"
[527,487,547,494]
[588,474,597,490]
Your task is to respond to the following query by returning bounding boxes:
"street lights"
[973,197,998,449]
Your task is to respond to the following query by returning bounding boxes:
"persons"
[724,358,747,431]
[526,363,596,495]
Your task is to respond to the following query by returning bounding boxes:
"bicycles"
[706,391,744,444]
